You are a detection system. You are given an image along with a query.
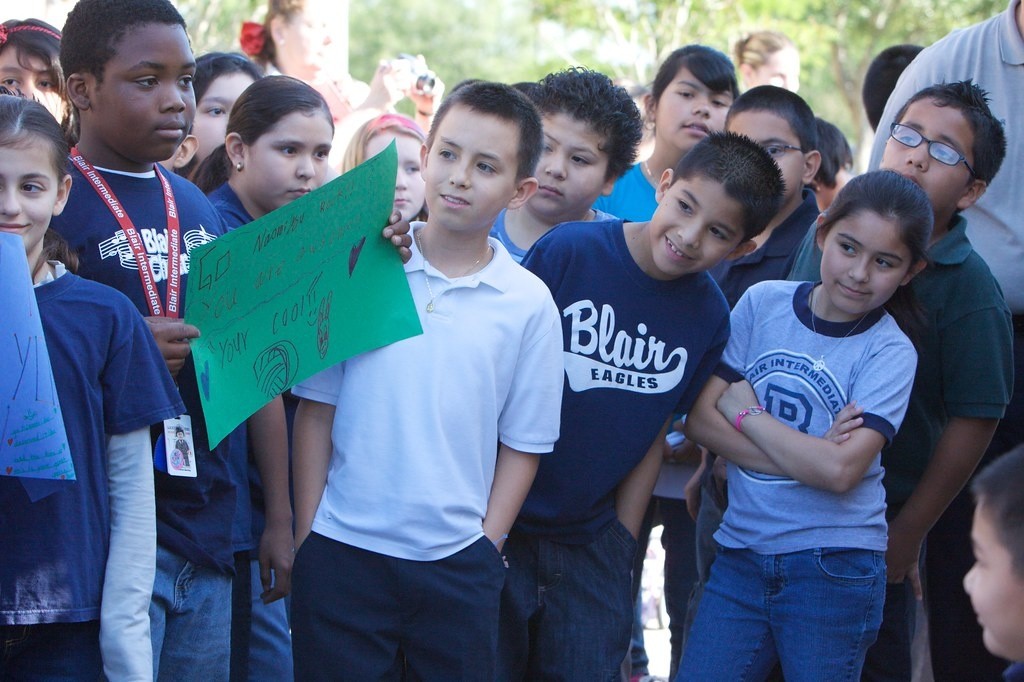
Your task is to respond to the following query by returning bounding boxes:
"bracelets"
[736,406,766,432]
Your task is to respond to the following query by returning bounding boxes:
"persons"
[43,1,413,682]
[499,132,787,682]
[1,95,186,682]
[675,168,933,682]
[293,80,564,682]
[0,0,1024,682]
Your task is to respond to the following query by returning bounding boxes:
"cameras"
[390,54,435,94]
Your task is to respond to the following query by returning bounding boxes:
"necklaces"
[645,159,661,187]
[811,296,872,372]
[417,231,490,275]
[416,230,490,312]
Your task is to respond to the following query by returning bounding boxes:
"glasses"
[763,145,807,158]
[890,122,977,177]
